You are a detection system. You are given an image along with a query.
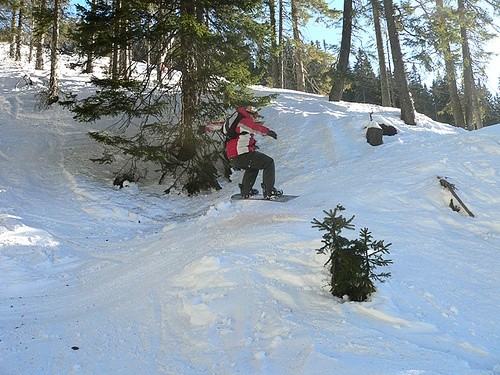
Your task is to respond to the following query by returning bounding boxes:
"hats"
[245,106,258,114]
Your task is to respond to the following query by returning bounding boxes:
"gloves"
[268,130,277,139]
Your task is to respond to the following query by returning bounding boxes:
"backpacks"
[222,110,249,140]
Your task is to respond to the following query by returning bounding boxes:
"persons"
[197,104,285,198]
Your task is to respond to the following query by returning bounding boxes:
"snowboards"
[231,194,299,203]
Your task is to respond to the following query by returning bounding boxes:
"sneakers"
[248,189,259,195]
[268,189,283,196]
